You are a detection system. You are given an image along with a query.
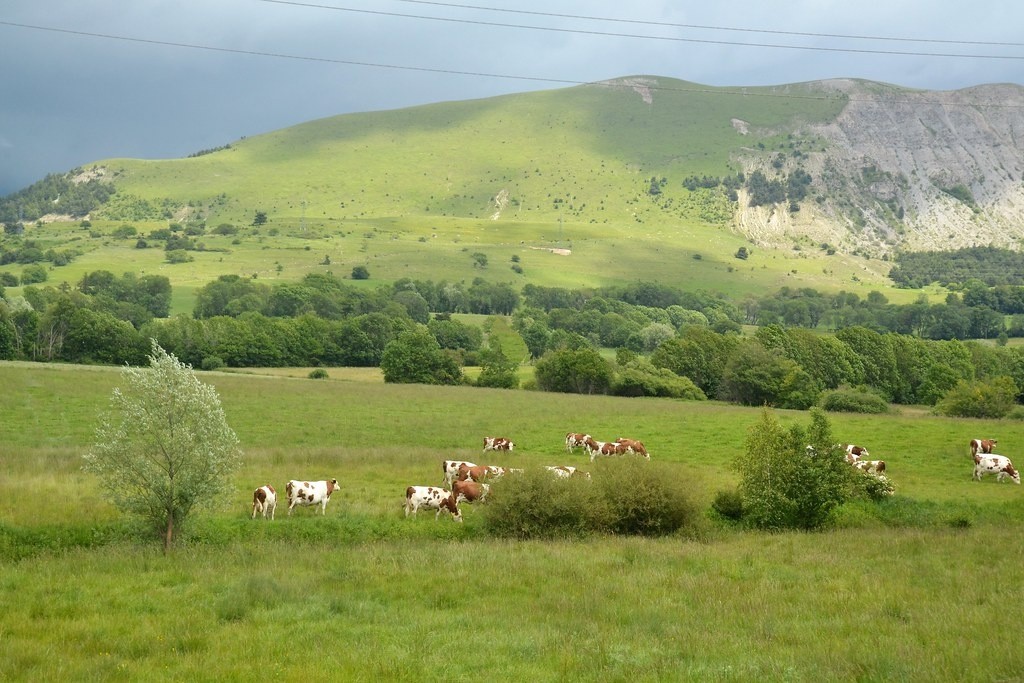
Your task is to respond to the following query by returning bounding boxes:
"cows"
[542,431,652,483]
[252,484,277,522]
[482,436,517,454]
[404,483,463,522]
[969,436,1021,486]
[442,459,525,506]
[285,476,341,517]
[805,442,896,499]
